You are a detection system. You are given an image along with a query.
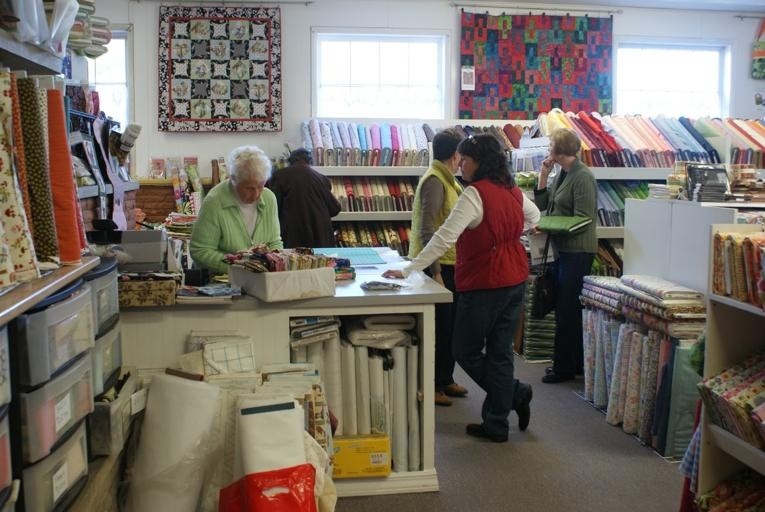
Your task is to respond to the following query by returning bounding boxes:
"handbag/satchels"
[532,268,558,317]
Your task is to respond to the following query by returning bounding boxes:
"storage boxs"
[0,259,127,511]
[228,261,337,303]
[116,280,177,308]
[328,431,392,479]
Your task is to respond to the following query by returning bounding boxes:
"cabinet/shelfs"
[582,166,683,274]
[617,190,764,303]
[694,222,765,510]
[0,15,106,331]
[307,159,430,262]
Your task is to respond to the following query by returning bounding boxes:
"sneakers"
[513,382,532,431]
[467,424,487,437]
[440,382,468,395]
[542,364,586,383]
[434,390,453,405]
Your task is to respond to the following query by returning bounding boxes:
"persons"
[188,145,283,283]
[382,135,532,442]
[268,147,341,246]
[533,128,597,383]
[407,131,468,405]
